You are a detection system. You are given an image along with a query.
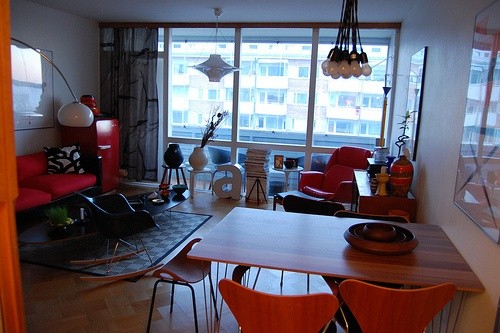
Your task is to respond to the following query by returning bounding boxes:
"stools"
[147,238,218,333]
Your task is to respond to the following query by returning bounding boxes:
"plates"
[152,199,164,203]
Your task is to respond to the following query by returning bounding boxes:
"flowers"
[201,106,231,148]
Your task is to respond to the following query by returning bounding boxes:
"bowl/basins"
[172,185,188,194]
[343,222,420,255]
[284,157,299,170]
[159,184,170,190]
[160,190,171,200]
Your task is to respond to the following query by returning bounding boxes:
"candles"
[381,166,387,174]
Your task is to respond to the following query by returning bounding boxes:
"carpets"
[19,212,213,282]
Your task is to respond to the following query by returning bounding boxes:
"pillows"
[43,143,86,174]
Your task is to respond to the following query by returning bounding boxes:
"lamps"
[10,37,94,127]
[187,7,242,82]
[321,0,372,79]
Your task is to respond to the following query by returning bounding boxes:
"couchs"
[14,153,103,226]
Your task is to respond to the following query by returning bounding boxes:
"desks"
[18,189,191,257]
[188,167,214,197]
[272,166,304,192]
[160,164,188,190]
[187,206,484,333]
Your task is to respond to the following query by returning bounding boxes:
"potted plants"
[45,206,75,232]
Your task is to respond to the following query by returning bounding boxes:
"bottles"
[163,143,184,168]
[390,154,414,197]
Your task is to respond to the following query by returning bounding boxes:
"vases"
[189,147,208,170]
[164,144,184,167]
[385,155,414,197]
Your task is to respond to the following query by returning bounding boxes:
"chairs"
[338,279,456,333]
[208,146,246,193]
[298,147,371,204]
[218,279,339,333]
[253,195,345,292]
[74,190,162,275]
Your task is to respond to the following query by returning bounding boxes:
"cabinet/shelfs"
[351,169,417,223]
